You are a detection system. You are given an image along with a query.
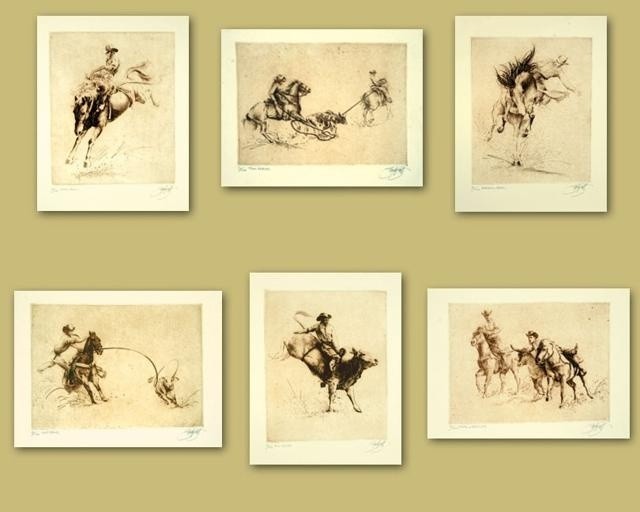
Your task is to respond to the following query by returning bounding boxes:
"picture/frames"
[249,272,404,466]
[427,288,633,441]
[222,28,426,189]
[11,290,224,449]
[454,14,609,213]
[36,15,191,212]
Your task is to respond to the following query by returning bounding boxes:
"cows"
[511,340,546,394]
[59,331,109,404]
[279,309,379,414]
[306,110,349,134]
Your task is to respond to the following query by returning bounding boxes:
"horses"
[467,327,523,396]
[534,338,597,405]
[241,78,312,144]
[484,44,546,168]
[65,60,161,168]
[361,78,395,124]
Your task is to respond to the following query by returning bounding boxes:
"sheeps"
[148,374,182,407]
